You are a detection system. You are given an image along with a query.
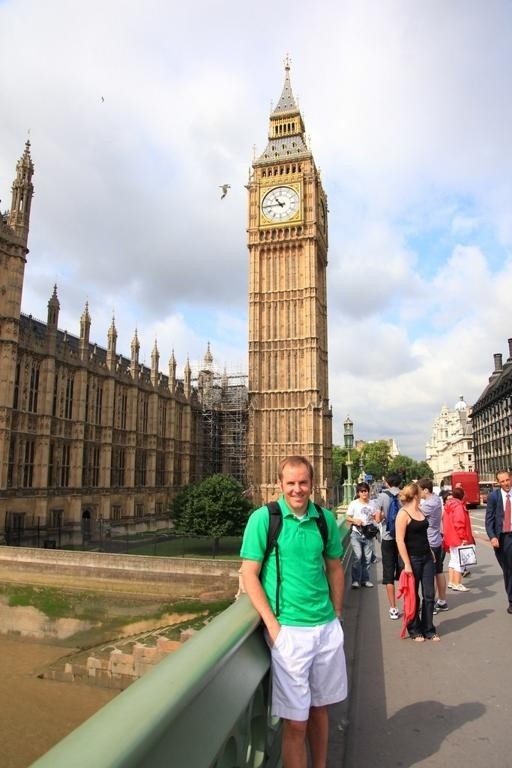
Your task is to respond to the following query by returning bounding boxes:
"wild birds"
[96,95,105,105]
[218,184,231,200]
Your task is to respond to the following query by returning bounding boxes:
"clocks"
[262,183,299,223]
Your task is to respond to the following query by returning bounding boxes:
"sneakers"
[352,570,470,642]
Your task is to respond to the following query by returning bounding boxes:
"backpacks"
[383,491,400,537]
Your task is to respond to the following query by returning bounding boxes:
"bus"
[478,480,499,501]
[439,471,481,508]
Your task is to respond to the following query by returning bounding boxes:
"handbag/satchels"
[457,542,478,568]
[354,524,379,539]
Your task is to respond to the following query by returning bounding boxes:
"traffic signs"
[365,474,373,480]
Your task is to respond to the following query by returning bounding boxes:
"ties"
[505,494,511,533]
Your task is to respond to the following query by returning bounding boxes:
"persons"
[441,486,475,592]
[415,478,448,615]
[395,481,441,642]
[484,470,511,614]
[345,482,378,588]
[375,472,405,621]
[240,458,349,767]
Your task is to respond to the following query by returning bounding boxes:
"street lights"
[358,454,365,473]
[342,415,357,506]
